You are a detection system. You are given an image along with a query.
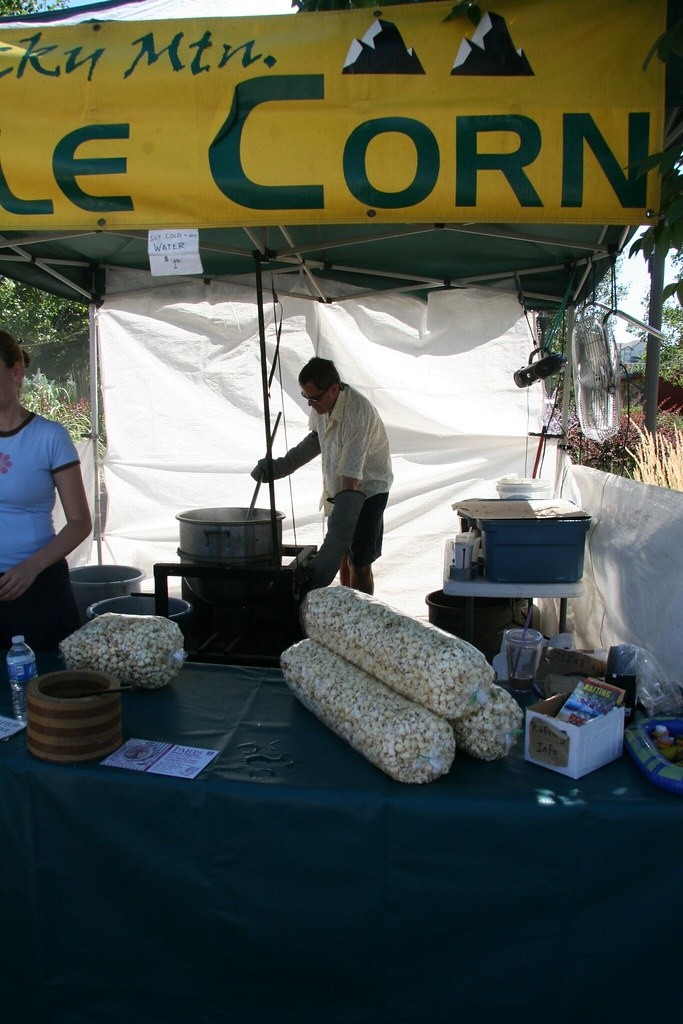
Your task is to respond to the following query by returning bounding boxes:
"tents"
[0,0,683,645]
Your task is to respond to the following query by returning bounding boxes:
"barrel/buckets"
[68,565,146,621]
[83,595,192,649]
[425,589,493,645]
[493,477,554,501]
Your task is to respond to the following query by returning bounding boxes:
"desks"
[0,659,683,1023]
[443,538,585,655]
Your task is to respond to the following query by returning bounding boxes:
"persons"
[250,357,393,596]
[0,330,92,648]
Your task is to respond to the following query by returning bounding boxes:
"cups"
[504,627,543,693]
[454,542,474,568]
[443,540,455,582]
[455,532,474,544]
[472,537,481,562]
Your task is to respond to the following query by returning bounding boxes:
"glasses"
[300,388,331,401]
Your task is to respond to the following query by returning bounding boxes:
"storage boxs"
[520,690,624,781]
[456,497,594,583]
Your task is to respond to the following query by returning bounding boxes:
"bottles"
[6,635,38,722]
[473,529,478,537]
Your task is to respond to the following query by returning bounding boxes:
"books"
[556,676,625,726]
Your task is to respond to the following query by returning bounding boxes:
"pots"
[176,506,285,598]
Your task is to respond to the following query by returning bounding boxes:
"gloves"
[251,430,321,482]
[302,490,367,594]
